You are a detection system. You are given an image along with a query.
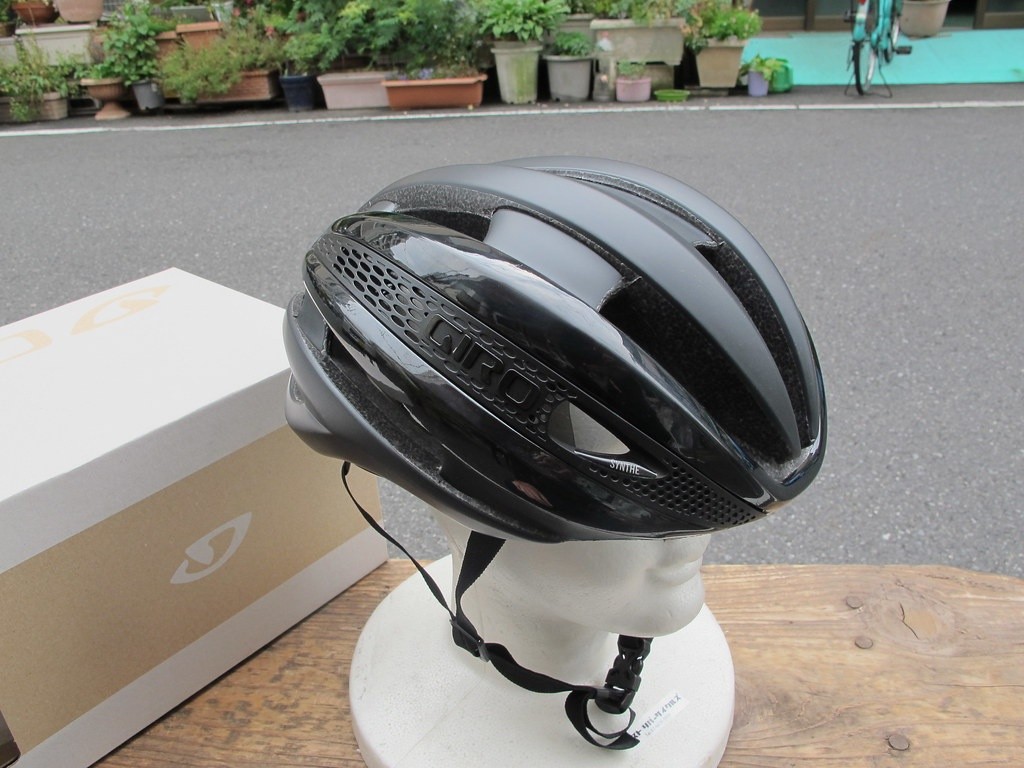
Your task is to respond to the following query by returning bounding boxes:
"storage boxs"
[0,267,390,768]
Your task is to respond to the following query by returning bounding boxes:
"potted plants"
[739,53,776,96]
[379,0,489,111]
[614,54,652,102]
[768,58,793,92]
[0,0,322,125]
[467,0,570,105]
[587,0,701,67]
[541,31,596,103]
[315,0,435,111]
[549,0,625,46]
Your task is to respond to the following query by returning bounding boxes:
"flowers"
[678,0,762,55]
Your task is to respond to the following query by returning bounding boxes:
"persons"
[283,154,825,768]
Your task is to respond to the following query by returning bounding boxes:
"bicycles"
[846,0,914,100]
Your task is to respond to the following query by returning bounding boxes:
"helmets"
[283,154,827,544]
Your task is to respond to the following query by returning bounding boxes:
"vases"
[898,0,951,37]
[694,43,747,88]
